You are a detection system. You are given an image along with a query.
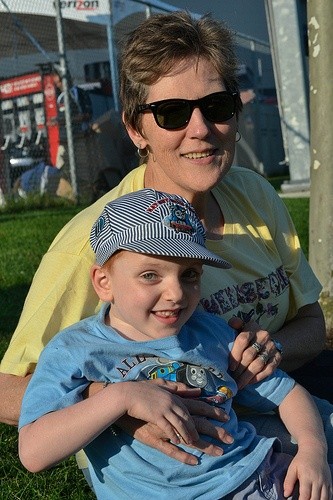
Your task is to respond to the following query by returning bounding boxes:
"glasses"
[135,88,242,131]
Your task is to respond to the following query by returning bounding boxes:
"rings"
[257,351,269,364]
[250,340,262,352]
[177,434,181,437]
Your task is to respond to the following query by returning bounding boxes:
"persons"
[16,189,333,500]
[0,9,333,496]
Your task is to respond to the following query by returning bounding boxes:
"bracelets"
[103,381,110,389]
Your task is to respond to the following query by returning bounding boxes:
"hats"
[89,189,233,269]
